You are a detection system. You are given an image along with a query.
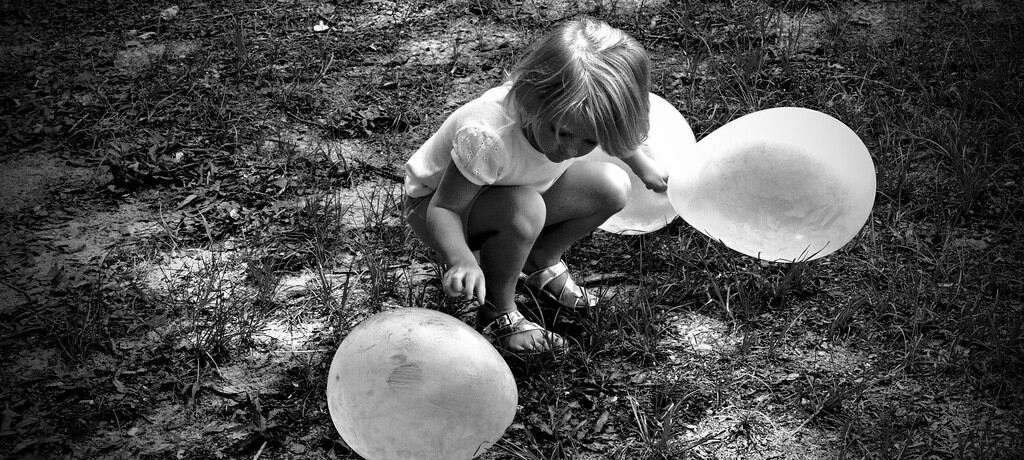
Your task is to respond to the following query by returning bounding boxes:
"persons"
[404,17,670,356]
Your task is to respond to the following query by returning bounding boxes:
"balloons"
[666,107,877,263]
[569,91,698,235]
[327,308,518,460]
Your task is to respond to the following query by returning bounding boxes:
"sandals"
[475,310,570,356]
[516,259,599,312]
